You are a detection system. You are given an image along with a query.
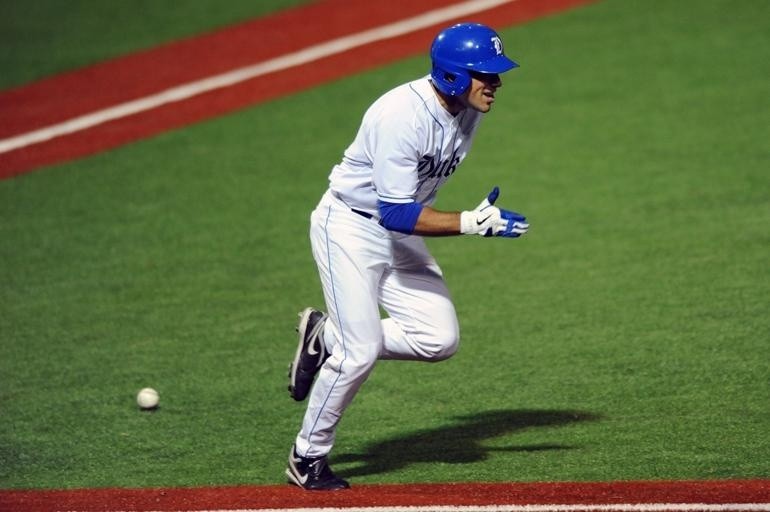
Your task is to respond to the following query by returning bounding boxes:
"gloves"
[458,187,531,240]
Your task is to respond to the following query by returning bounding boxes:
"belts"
[350,208,375,220]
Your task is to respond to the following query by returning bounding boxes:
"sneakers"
[284,444,351,493]
[284,306,330,404]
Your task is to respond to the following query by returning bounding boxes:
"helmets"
[429,22,520,100]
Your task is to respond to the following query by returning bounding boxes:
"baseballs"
[137,388,160,409]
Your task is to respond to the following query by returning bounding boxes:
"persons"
[285,21,529,490]
